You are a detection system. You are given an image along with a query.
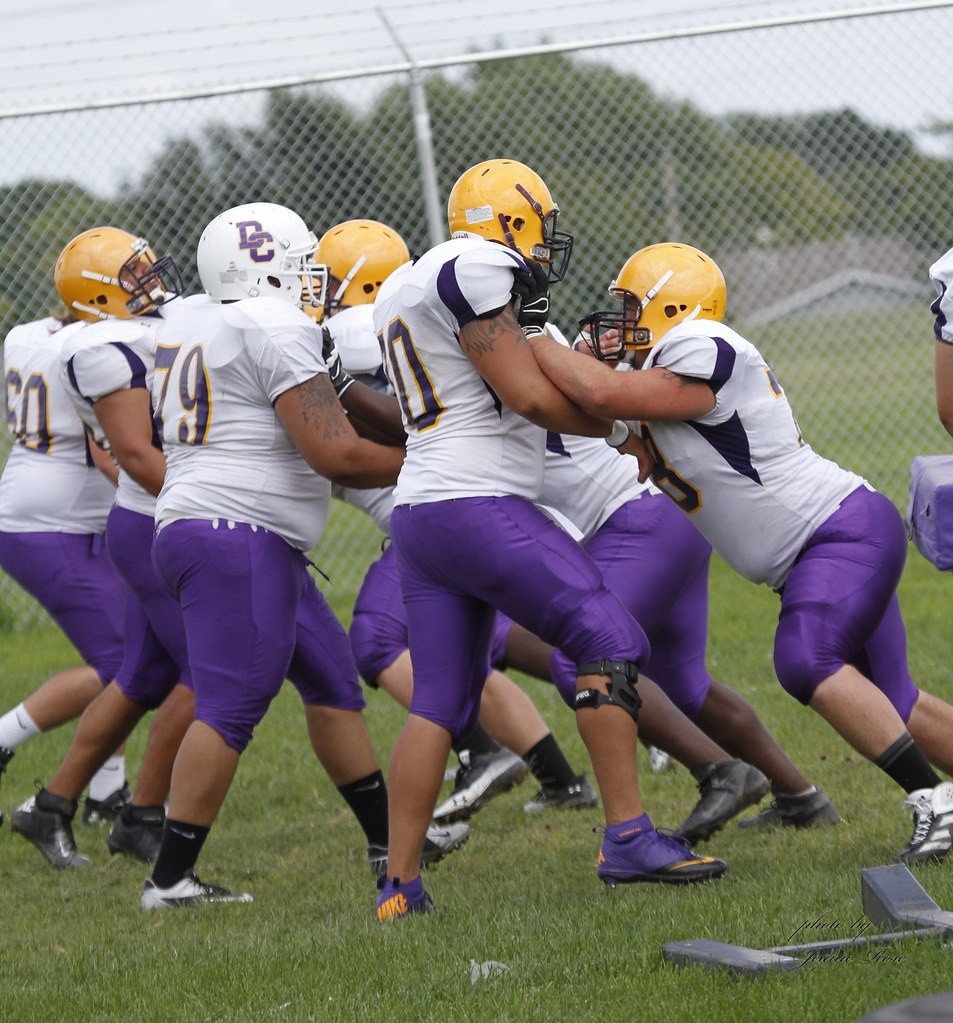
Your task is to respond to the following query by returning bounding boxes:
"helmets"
[579,243,727,350]
[448,158,574,286]
[54,226,184,319]
[196,202,329,306]
[315,219,412,312]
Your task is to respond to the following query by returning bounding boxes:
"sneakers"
[899,790,953,863]
[12,788,93,870]
[107,806,166,865]
[671,761,769,843]
[735,791,839,831]
[377,893,441,920]
[366,822,470,878]
[140,876,255,908]
[433,749,528,826]
[594,815,728,883]
[522,774,600,810]
[82,784,134,826]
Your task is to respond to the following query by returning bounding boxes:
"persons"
[0,160,848,923]
[929,246,953,439]
[510,245,953,865]
